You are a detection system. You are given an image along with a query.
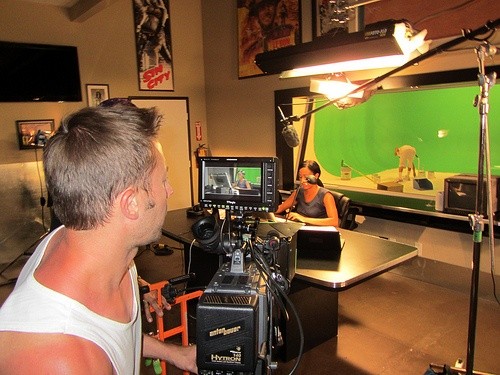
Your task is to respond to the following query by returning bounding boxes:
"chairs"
[329,191,353,230]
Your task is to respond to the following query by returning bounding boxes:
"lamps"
[254,20,426,81]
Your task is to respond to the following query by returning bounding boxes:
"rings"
[143,301,148,304]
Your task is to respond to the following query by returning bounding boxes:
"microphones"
[277,106,300,148]
[267,230,280,248]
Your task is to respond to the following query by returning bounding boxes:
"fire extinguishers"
[196,143,208,168]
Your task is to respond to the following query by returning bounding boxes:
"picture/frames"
[236,0,303,80]
[15,119,55,149]
[132,0,174,92]
[86,84,110,108]
[311,0,365,41]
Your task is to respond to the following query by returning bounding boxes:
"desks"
[157,207,419,365]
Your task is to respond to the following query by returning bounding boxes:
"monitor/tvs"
[0,41,83,102]
[199,156,280,212]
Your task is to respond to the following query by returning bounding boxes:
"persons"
[235,171,251,189]
[395,145,417,183]
[138,275,171,322]
[0,98,199,375]
[275,160,338,226]
[33,129,43,146]
